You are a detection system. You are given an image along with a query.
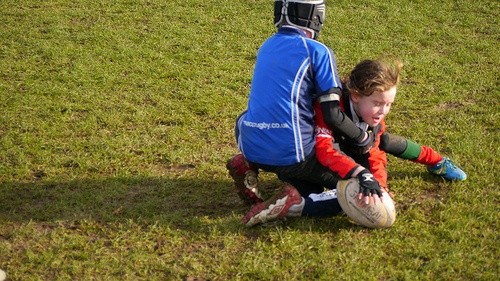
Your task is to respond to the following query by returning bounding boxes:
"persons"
[314,59,469,205]
[228,2,376,231]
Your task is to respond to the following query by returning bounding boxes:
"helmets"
[273,1,325,38]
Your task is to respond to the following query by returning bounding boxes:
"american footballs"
[336,178,396,228]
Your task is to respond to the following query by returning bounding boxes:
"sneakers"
[427,155,468,181]
[242,183,301,227]
[225,154,266,204]
